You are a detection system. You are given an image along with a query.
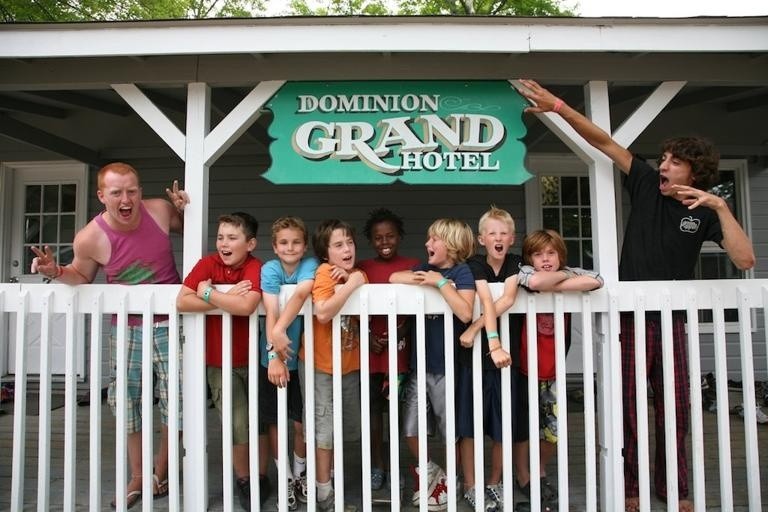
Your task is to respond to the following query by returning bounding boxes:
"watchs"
[265,342,274,351]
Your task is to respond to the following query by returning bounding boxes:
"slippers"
[111,490,142,509]
[514,474,558,512]
[153,465,168,500]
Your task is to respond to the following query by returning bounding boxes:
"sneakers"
[484,478,504,512]
[428,472,462,512]
[408,457,444,507]
[386,469,407,490]
[464,483,498,512]
[260,474,271,505]
[371,466,386,490]
[237,476,250,512]
[701,371,768,424]
[275,476,297,510]
[316,486,358,512]
[293,472,307,504]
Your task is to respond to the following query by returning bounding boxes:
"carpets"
[0,392,84,416]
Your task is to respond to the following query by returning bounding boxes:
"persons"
[29,162,191,511]
[457,206,530,511]
[257,217,323,512]
[175,211,272,511]
[354,207,424,499]
[516,229,606,504]
[516,75,757,512]
[308,219,371,512]
[388,217,477,511]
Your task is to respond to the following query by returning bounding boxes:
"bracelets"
[53,266,63,279]
[485,332,500,339]
[435,276,449,289]
[551,98,563,112]
[201,286,214,304]
[267,353,281,359]
[489,345,502,352]
